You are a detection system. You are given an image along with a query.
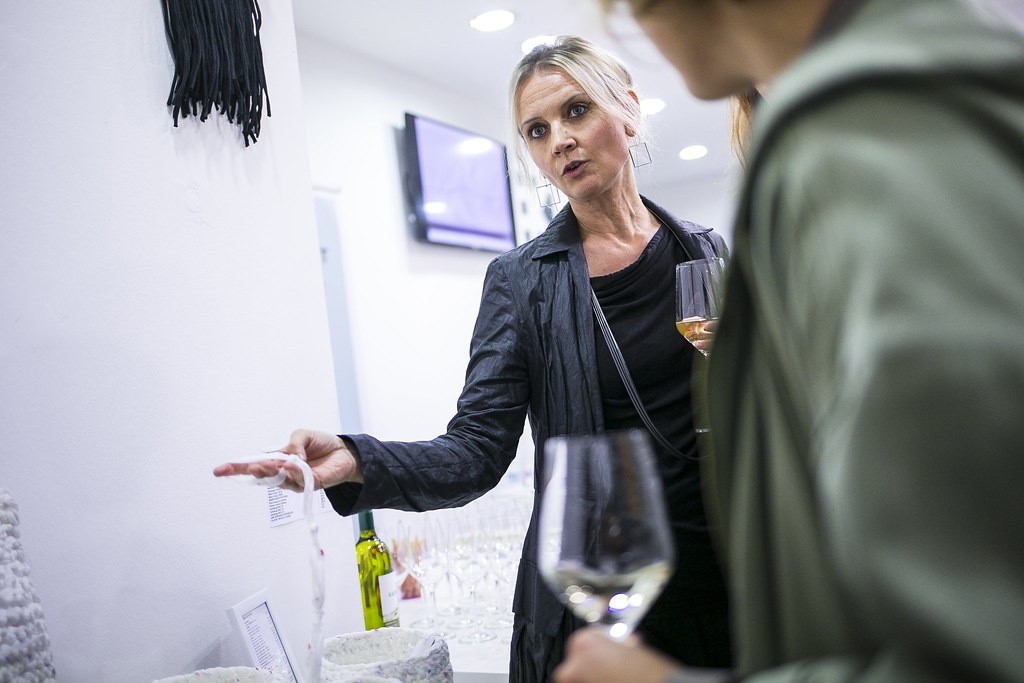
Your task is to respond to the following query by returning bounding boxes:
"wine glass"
[675,257,731,433]
[397,493,532,642]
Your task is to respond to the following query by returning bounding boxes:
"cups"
[536,429,676,645]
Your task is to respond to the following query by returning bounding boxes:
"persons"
[557,0,1024,682]
[212,37,729,683]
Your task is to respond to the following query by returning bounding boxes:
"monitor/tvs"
[406,112,517,254]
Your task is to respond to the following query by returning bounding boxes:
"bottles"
[355,509,400,631]
